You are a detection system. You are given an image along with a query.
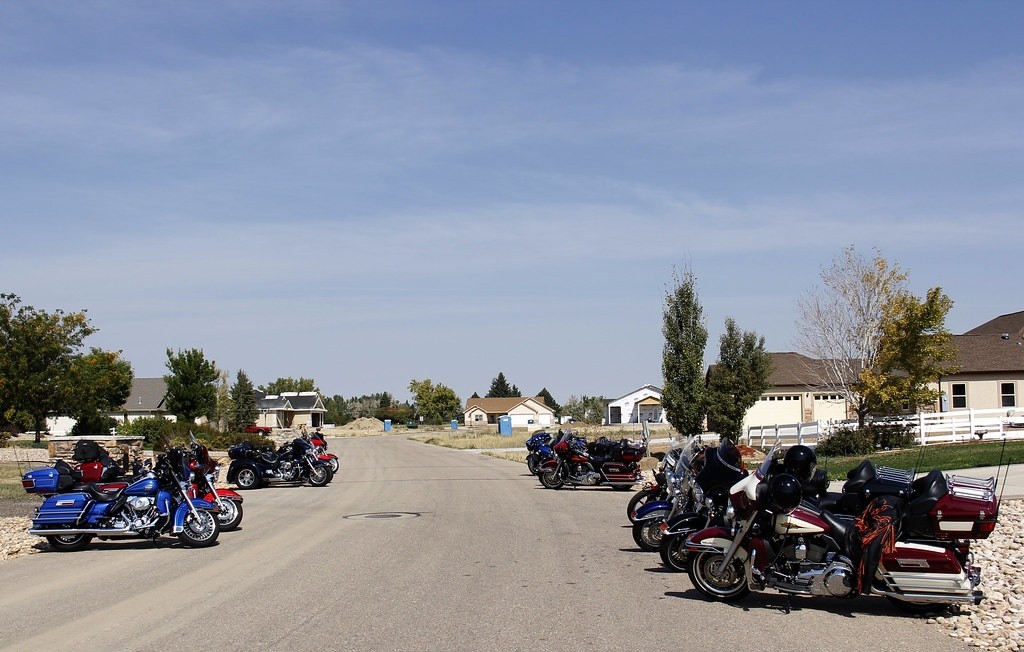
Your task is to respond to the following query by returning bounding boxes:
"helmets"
[785,445,817,478]
[768,474,802,510]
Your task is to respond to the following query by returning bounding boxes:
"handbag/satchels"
[73,439,98,459]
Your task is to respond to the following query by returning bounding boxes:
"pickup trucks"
[229,420,273,436]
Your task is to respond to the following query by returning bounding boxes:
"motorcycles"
[525,428,648,491]
[22,429,244,552]
[227,426,339,490]
[628,429,1011,615]
